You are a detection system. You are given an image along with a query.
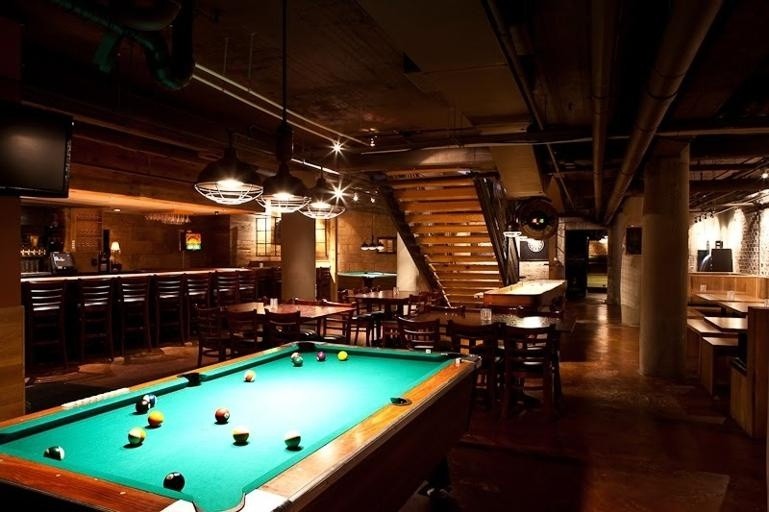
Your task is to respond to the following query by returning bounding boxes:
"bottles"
[705,240,710,249]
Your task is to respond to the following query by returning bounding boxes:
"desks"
[0,340,485,511]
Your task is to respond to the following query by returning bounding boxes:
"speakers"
[626,227,642,254]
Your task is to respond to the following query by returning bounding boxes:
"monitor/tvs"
[180,232,202,251]
[50,251,75,270]
[0,96,73,198]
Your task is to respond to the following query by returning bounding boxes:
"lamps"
[693,207,714,223]
[191,0,264,206]
[253,0,312,213]
[361,195,385,252]
[300,0,347,221]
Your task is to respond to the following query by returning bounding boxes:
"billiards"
[291,351,348,367]
[44,445,65,461]
[234,427,250,441]
[136,393,157,414]
[147,410,163,426]
[163,473,184,491]
[128,428,145,444]
[244,371,256,382]
[215,408,230,424]
[283,431,300,447]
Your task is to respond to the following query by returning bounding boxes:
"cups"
[699,284,708,293]
[763,298,769,309]
[270,298,278,309]
[481,308,493,321]
[392,286,399,295]
[726,290,736,300]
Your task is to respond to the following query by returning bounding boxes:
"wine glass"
[144,211,193,226]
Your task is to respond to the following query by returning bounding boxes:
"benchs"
[679,274,769,442]
